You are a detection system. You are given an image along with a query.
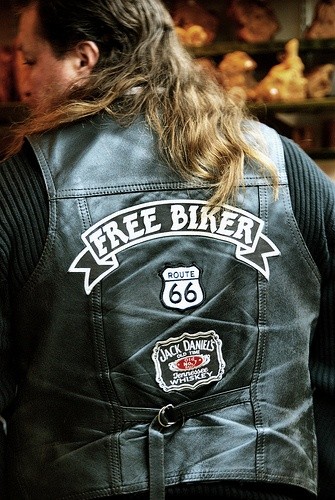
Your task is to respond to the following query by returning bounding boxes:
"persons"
[0,0,335,500]
